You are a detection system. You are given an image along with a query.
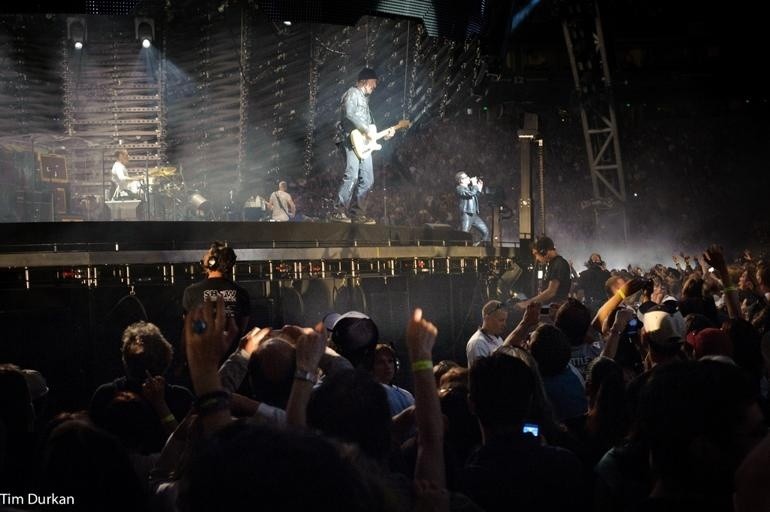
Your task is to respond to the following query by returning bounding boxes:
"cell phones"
[521,422,539,438]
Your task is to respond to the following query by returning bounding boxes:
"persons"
[269,181,296,222]
[110,149,146,219]
[0,235,770,512]
[455,171,489,246]
[331,67,396,225]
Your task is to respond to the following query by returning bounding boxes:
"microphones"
[199,259,209,270]
[470,176,486,180]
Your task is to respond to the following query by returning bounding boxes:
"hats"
[323,311,378,351]
[359,68,376,79]
[637,301,685,346]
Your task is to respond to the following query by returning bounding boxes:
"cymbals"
[148,166,178,177]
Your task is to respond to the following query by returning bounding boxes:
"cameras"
[627,317,640,329]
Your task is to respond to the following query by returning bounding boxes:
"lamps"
[134,9,155,49]
[66,14,91,50]
[191,193,211,213]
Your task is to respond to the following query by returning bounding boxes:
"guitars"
[351,119,411,161]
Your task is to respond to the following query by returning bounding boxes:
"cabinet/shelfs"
[16,187,66,222]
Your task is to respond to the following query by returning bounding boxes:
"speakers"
[425,222,452,245]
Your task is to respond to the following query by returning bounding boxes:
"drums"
[160,181,176,193]
[141,184,159,193]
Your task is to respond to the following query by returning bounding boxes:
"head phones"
[208,256,218,269]
[540,241,547,256]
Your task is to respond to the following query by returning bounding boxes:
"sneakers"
[331,212,376,224]
